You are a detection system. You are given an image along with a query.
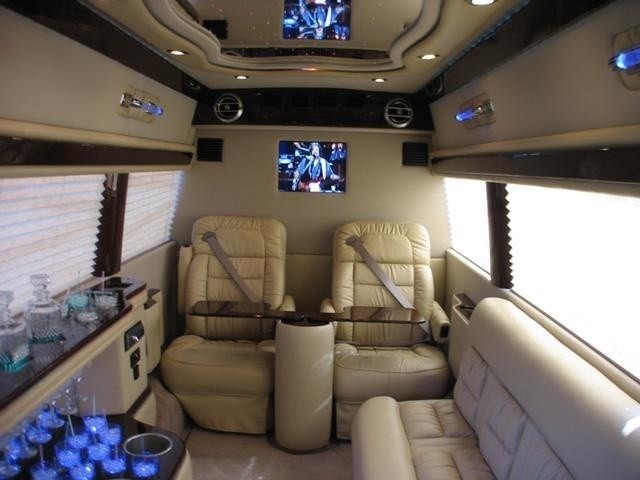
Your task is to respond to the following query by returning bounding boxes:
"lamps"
[318,221,452,441]
[160,214,297,437]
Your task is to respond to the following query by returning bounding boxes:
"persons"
[336,142,346,176]
[293,140,311,160]
[292,141,339,192]
[290,0,349,41]
[329,143,338,173]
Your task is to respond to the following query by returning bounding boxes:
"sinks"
[349,295,640,479]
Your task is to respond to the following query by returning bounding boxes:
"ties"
[276,138,348,194]
[281,0,353,41]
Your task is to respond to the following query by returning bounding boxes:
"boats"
[195,135,225,163]
[402,140,431,168]
[201,18,230,41]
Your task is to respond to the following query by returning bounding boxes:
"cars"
[68,290,118,311]
[122,432,173,480]
